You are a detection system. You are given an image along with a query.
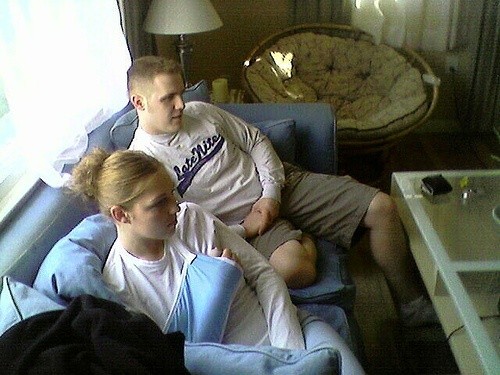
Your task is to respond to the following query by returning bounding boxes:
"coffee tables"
[390,169,500,375]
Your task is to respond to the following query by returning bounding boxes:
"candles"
[212,78,232,105]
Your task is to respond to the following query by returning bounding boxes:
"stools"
[241,22,442,189]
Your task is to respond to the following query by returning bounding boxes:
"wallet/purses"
[420,172,453,196]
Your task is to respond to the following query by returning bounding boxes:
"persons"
[123,55,413,325]
[73,147,369,375]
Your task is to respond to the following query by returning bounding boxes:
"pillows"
[247,118,298,167]
[108,79,212,152]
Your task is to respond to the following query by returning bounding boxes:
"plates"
[491,205,500,224]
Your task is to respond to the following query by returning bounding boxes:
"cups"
[213,78,229,103]
[458,189,475,209]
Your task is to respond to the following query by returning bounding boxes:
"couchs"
[0,79,370,375]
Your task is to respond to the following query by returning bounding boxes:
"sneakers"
[398,295,441,327]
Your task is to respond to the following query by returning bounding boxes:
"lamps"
[142,0,225,91]
